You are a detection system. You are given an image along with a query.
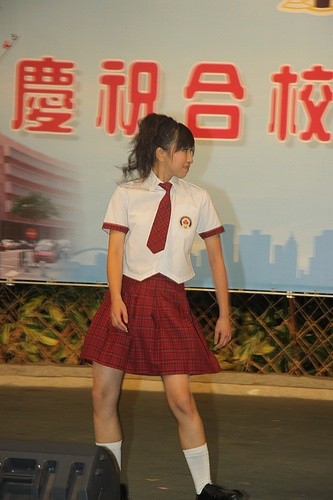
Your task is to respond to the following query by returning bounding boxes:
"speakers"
[0,439,120,500]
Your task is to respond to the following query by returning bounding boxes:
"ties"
[147,182,173,254]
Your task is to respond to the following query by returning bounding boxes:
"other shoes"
[195,482,242,500]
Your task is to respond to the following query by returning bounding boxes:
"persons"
[79,112,243,500]
